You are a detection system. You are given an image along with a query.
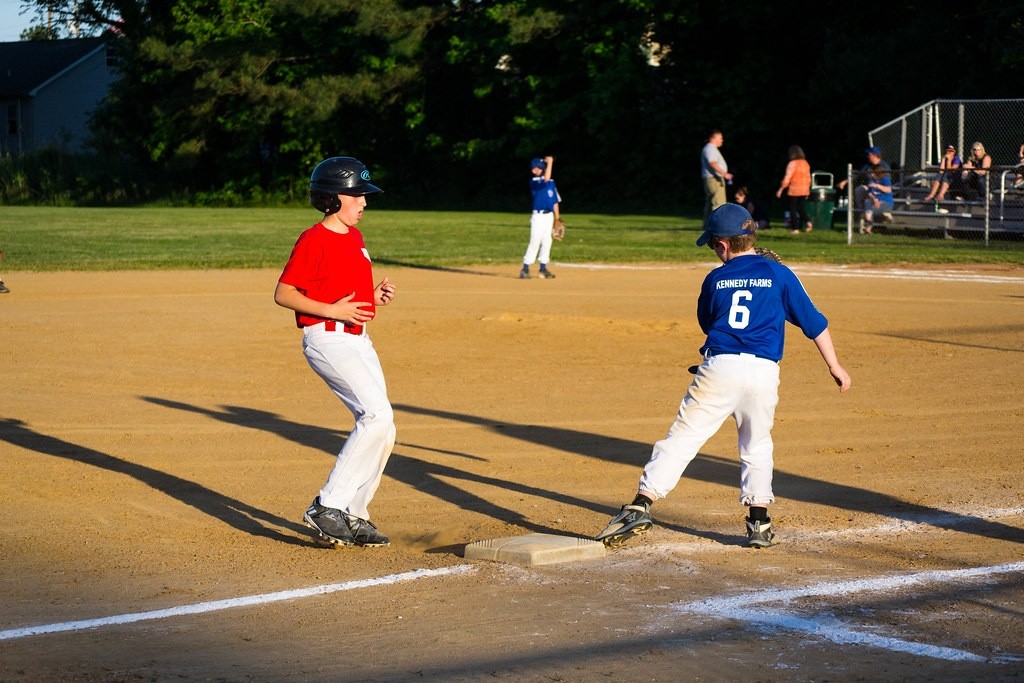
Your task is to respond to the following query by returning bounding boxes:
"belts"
[324,321,364,335]
[537,210,550,213]
[711,344,780,362]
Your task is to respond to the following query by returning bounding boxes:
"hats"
[533,158,547,169]
[695,204,757,246]
[946,145,955,151]
[866,147,880,155]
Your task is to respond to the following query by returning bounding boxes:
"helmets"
[309,156,382,216]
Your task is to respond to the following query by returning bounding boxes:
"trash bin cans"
[803,170,836,229]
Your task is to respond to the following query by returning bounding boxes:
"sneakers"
[304,496,355,549]
[520,272,531,280]
[744,515,777,547]
[342,511,390,548]
[595,503,654,550]
[539,268,555,278]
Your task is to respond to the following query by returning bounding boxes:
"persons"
[597,202,852,547]
[274,157,397,549]
[733,185,772,230]
[838,147,893,235]
[924,145,962,200]
[1012,144,1024,188]
[519,156,566,279]
[699,132,733,212]
[962,142,992,200]
[775,147,813,234]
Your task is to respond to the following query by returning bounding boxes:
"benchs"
[850,171,1024,222]
